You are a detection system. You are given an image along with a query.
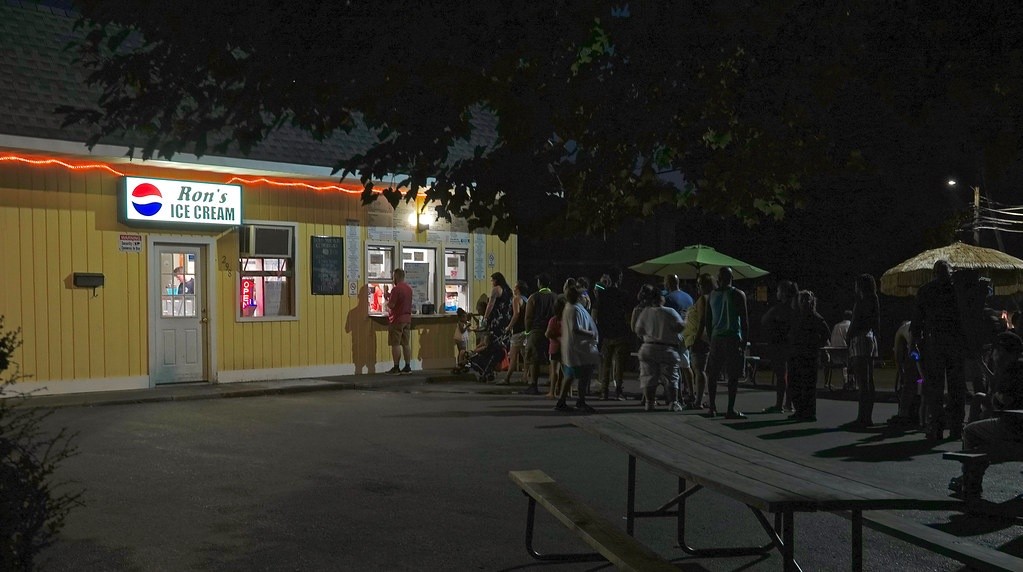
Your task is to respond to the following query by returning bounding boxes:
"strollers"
[452,330,512,383]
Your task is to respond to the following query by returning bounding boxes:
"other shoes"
[573,401,596,412]
[615,393,627,401]
[669,401,682,411]
[555,400,572,411]
[546,393,555,399]
[683,402,703,410]
[521,386,538,394]
[788,414,817,422]
[726,409,746,419]
[645,402,654,411]
[708,410,717,417]
[762,405,785,413]
[496,379,510,385]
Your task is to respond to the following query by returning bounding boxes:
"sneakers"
[385,367,401,375]
[401,367,412,375]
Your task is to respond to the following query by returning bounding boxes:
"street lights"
[947,171,981,247]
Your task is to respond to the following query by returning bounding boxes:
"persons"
[174,267,194,294]
[454,260,1023,500]
[386,269,413,374]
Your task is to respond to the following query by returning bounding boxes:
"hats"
[994,331,1023,354]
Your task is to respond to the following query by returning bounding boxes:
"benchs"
[715,341,851,390]
[508,469,689,572]
[831,509,1023,572]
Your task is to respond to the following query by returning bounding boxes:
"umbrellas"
[880,239,1022,296]
[626,243,771,296]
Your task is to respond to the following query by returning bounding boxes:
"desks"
[568,413,965,572]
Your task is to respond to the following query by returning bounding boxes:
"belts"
[647,341,678,348]
